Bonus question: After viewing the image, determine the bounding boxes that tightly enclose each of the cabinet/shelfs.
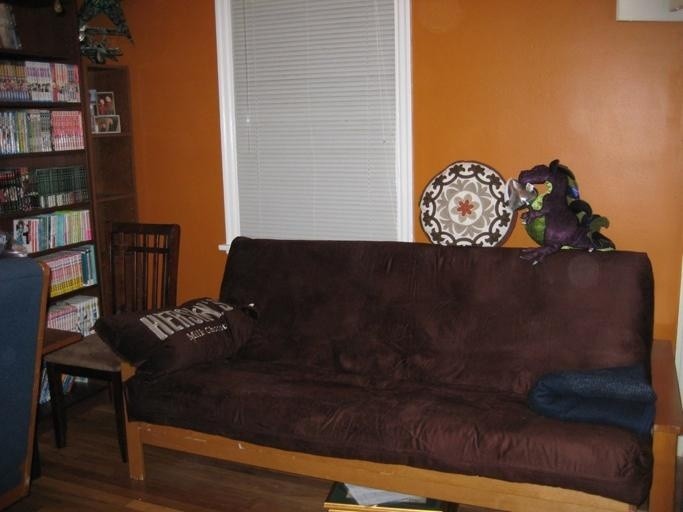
[0,49,109,424]
[83,60,147,385]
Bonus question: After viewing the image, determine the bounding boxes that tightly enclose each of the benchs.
[117,236,683,512]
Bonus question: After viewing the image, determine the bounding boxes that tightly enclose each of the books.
[0,59,103,404]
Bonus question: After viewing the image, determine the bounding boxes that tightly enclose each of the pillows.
[94,294,267,381]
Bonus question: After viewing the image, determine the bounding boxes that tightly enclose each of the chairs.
[42,221,180,448]
[1,253,52,511]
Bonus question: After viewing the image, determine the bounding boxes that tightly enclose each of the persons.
[99,95,115,131]
